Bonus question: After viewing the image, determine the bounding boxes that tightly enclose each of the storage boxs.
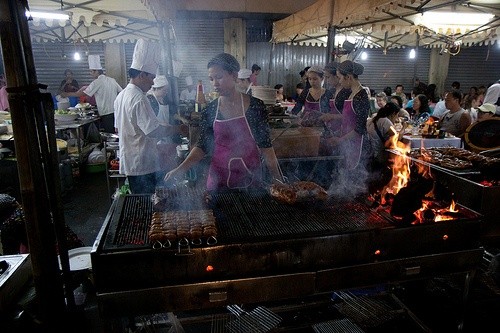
[269,127,324,159]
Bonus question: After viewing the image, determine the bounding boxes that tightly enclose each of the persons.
[59,69,81,93]
[180,75,199,103]
[274,83,285,102]
[250,64,262,86]
[289,66,329,121]
[300,62,348,157]
[318,59,371,184]
[358,77,500,183]
[63,54,127,152]
[165,52,284,192]
[236,68,256,96]
[146,73,194,145]
[115,37,192,195]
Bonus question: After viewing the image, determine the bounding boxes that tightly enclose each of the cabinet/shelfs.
[54,117,105,186]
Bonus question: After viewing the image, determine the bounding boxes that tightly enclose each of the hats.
[88,54,102,70]
[186,75,193,86]
[474,102,496,114]
[130,38,160,75]
[151,74,168,87]
[237,67,252,78]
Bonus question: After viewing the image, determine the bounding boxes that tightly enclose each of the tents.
[27,1,178,92]
[272,0,500,90]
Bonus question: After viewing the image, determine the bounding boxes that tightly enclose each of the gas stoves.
[0,253,34,321]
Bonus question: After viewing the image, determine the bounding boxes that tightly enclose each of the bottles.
[412,118,420,136]
[194,80,206,113]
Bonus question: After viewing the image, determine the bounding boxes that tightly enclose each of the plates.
[57,247,93,270]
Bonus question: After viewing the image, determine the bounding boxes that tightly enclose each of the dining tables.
[392,123,462,150]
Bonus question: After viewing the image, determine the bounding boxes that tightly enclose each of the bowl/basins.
[99,132,118,141]
[54,114,79,121]
[88,162,107,172]
[268,106,286,116]
